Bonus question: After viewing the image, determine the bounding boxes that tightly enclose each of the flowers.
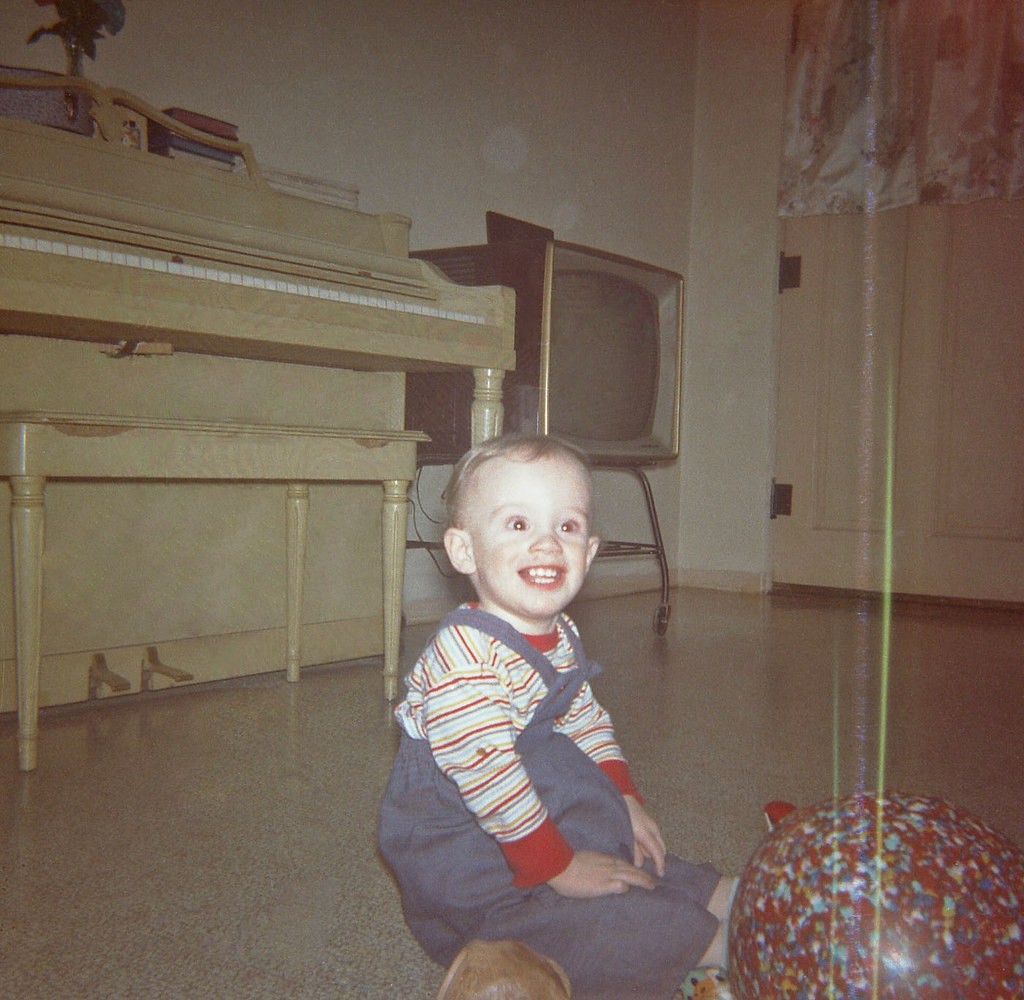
[28,0,127,60]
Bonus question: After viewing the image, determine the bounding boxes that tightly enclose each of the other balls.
[722,787,1023,1000]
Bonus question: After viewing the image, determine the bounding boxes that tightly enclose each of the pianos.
[0,71,517,773]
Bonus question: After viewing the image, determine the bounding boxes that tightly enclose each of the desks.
[401,454,671,637]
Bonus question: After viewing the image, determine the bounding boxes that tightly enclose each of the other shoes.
[762,800,796,831]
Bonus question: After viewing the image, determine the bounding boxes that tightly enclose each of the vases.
[63,42,88,77]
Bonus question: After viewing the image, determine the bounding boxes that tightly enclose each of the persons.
[372,431,738,1000]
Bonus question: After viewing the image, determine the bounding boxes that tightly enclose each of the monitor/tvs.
[405,238,686,462]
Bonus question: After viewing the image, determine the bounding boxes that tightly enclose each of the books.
[147,107,239,174]
[254,164,361,212]
